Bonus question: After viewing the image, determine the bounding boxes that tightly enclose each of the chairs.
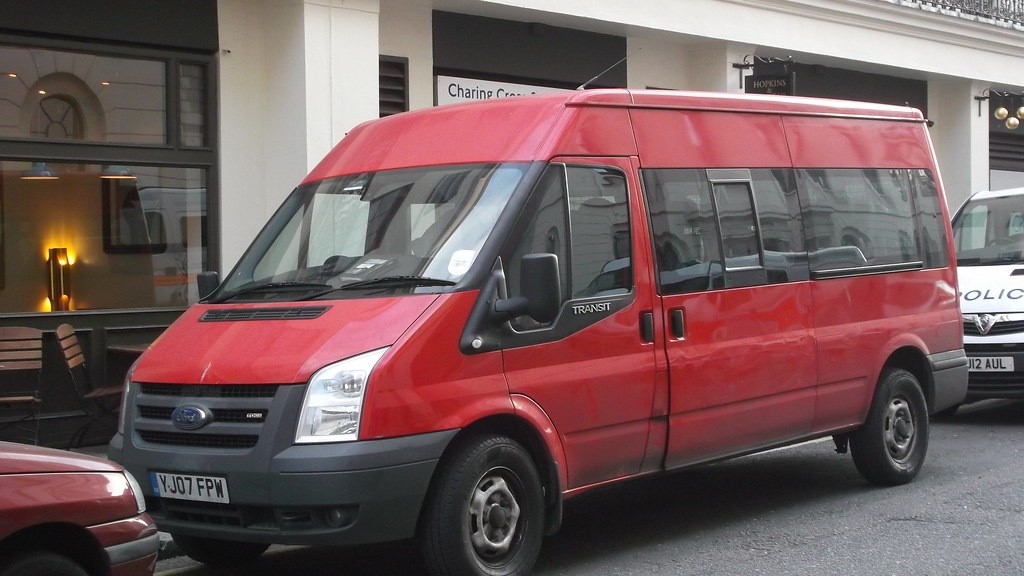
[0,327,43,445]
[574,196,617,290]
[55,323,124,451]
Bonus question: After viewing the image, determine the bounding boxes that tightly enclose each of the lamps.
[18,163,61,180]
[101,163,137,180]
[974,87,1024,130]
[47,247,72,311]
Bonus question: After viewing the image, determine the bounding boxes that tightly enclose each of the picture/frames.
[102,163,167,254]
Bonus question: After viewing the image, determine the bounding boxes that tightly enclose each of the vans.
[941,187,1024,423]
[104,85,974,576]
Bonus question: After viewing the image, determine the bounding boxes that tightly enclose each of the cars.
[0,439,162,576]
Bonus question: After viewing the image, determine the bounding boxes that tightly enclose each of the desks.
[109,344,148,354]
